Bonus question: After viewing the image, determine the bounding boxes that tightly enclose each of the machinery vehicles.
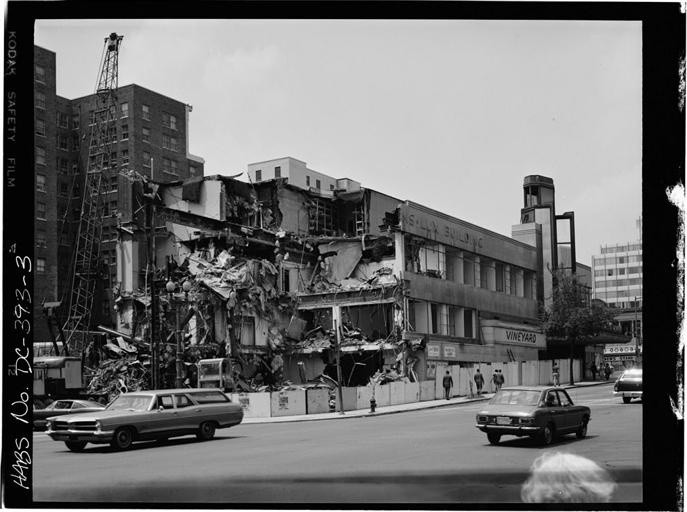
[33,33,123,403]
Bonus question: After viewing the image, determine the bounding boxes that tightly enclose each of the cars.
[47,386,245,453]
[474,384,592,448]
[32,398,106,434]
[612,370,643,404]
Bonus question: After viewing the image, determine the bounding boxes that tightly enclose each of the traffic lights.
[329,331,336,354]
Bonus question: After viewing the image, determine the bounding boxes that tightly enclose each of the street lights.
[164,279,193,391]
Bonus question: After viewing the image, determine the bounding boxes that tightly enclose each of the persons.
[474,369,484,394]
[591,361,611,381]
[442,370,453,400]
[552,363,560,387]
[490,369,504,392]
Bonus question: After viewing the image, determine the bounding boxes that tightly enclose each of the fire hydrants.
[369,398,377,412]
